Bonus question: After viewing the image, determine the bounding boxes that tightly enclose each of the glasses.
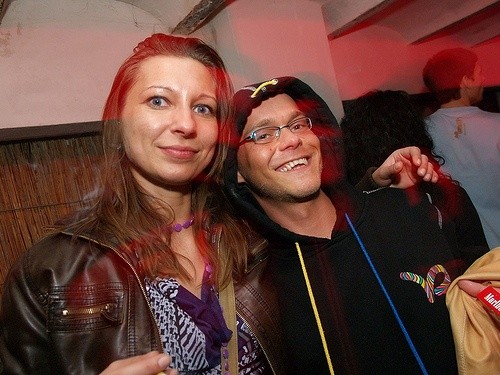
[236,116,312,149]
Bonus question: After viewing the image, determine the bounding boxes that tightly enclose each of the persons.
[224,76,500,375]
[0,34,438,375]
[423,49,500,250]
[339,88,445,172]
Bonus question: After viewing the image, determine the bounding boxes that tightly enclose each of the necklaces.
[172,219,194,232]
